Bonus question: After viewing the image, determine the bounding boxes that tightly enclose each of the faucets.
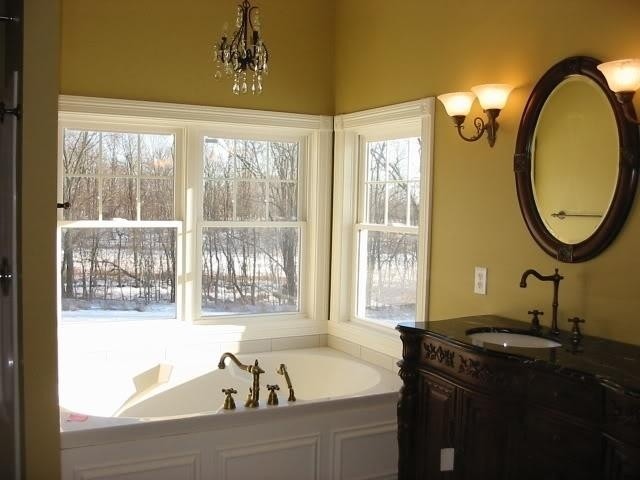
[218,353,265,408]
[277,364,296,402]
[519,268,565,334]
[466,327,563,349]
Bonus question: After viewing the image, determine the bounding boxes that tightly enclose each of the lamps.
[208,0,272,96]
[437,84,513,148]
[597,58,640,124]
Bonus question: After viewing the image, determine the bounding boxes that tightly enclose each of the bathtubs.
[57,347,404,480]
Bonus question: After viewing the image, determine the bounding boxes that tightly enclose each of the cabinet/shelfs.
[397,326,640,480]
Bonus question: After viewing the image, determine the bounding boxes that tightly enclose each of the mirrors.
[514,56,639,264]
